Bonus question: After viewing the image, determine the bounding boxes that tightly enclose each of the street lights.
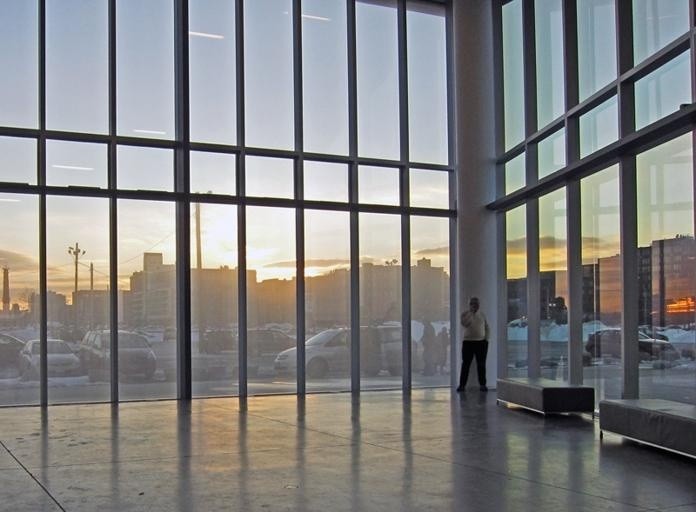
[385,258,398,266]
[68,243,86,320]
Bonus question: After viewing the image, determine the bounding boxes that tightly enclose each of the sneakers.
[480,386,488,392]
[457,386,464,392]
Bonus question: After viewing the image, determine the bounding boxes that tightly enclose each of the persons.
[436,326,450,375]
[547,295,570,382]
[456,297,491,392]
[420,319,437,377]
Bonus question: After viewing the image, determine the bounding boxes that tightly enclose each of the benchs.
[496,377,595,419]
[599,399,696,459]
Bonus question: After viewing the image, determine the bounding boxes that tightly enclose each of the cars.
[585,326,676,360]
